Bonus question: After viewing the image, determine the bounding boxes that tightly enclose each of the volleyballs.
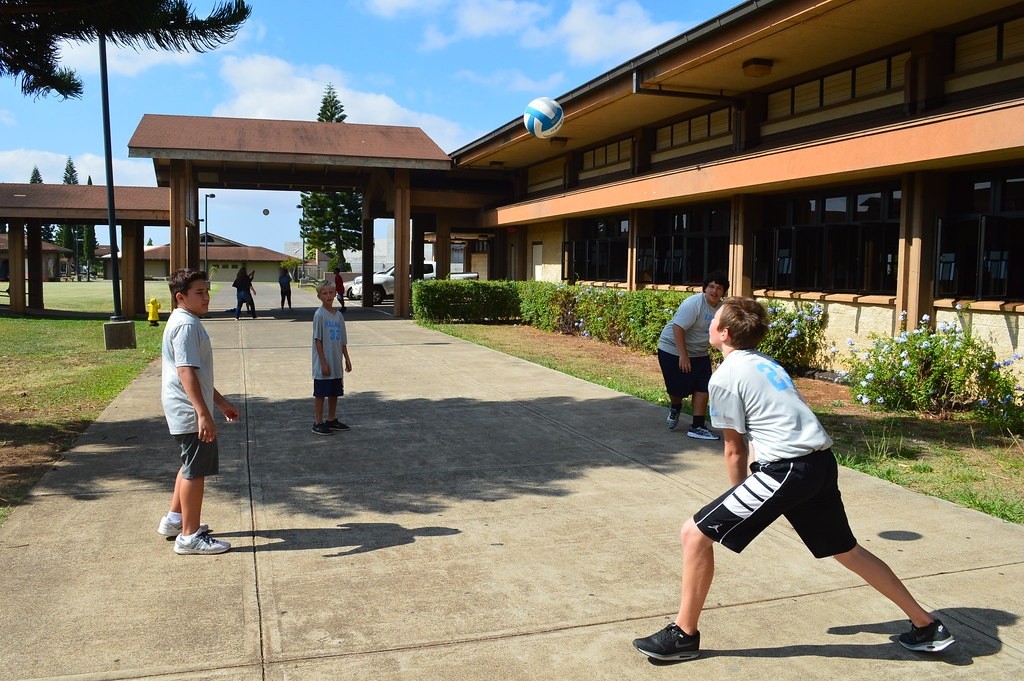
[523,97,565,140]
[263,209,269,215]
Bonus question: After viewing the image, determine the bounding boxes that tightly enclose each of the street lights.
[204,193,215,291]
[296,204,305,279]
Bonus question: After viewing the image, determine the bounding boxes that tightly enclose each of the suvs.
[339,259,436,306]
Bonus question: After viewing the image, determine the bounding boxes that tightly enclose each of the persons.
[235,265,257,321]
[310,280,352,435]
[278,266,293,311]
[657,272,730,440]
[333,267,347,313]
[156,267,239,554]
[632,293,955,662]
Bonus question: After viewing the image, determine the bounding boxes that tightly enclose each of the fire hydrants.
[146,297,161,326]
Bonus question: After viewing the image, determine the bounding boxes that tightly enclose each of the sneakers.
[312,422,334,435]
[173,528,231,554]
[326,418,350,431]
[666,403,682,430]
[633,621,701,660]
[687,425,720,440]
[899,619,956,652]
[158,516,208,536]
